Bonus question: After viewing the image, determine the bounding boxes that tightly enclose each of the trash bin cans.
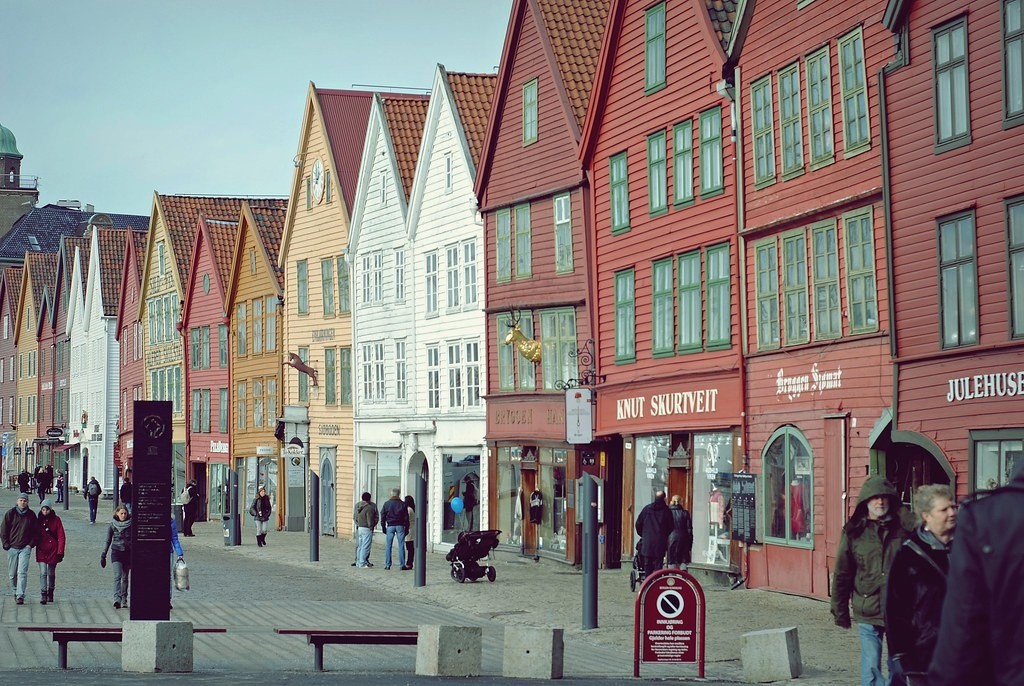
[223,514,242,545]
[170,504,184,532]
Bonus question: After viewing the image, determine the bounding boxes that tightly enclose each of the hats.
[40,498,53,509]
[17,493,29,500]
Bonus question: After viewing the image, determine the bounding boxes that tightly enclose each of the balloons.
[451,497,464,514]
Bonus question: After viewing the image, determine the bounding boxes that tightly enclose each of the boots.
[255,533,267,547]
[47,587,55,602]
[40,591,48,604]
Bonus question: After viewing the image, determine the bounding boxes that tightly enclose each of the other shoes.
[384,567,390,570]
[122,603,127,608]
[405,564,412,569]
[114,600,121,609]
[16,598,24,605]
[400,566,409,570]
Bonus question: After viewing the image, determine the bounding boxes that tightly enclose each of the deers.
[503,306,541,363]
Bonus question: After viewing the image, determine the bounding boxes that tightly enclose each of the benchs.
[18,626,227,669]
[272,626,418,673]
[103,487,121,499]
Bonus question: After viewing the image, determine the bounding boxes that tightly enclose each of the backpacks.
[88,484,98,496]
[181,486,193,504]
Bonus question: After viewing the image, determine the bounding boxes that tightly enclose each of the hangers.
[530,487,544,497]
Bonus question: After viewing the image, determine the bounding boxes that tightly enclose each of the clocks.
[311,156,325,205]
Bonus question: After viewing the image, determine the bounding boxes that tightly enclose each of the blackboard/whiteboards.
[731,473,761,546]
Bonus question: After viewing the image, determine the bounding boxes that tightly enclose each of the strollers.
[450,529,502,583]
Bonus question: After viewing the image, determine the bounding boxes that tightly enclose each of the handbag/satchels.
[173,557,190,591]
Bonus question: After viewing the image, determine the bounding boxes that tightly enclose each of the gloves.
[894,658,906,685]
[382,527,387,534]
[404,529,409,535]
[100,553,106,568]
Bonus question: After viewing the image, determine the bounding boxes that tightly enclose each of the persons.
[668,495,693,580]
[249,488,272,547]
[886,484,957,686]
[710,488,724,537]
[18,465,64,504]
[101,478,183,609]
[780,480,810,540]
[831,476,916,686]
[84,477,102,524]
[635,490,674,588]
[0,493,37,604]
[32,499,66,604]
[351,488,416,570]
[926,462,1024,686]
[462,475,476,533]
[180,479,199,536]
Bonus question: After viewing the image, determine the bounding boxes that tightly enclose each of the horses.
[281,353,318,386]
